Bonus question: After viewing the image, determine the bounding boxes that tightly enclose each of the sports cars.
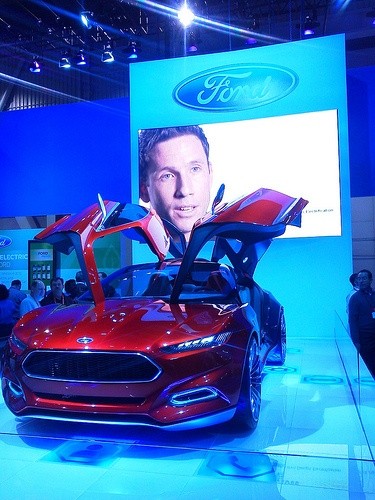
[2,188,287,431]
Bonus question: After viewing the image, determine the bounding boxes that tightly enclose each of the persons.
[65,270,107,299]
[0,284,16,328]
[40,277,74,305]
[345,269,375,376]
[7,280,27,304]
[138,125,211,242]
[20,280,45,317]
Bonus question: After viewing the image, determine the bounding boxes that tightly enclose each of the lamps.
[304,15,315,35]
[187,30,199,52]
[127,41,138,59]
[29,55,41,73]
[76,48,86,65]
[245,14,258,44]
[58,49,71,69]
[101,42,114,63]
[80,11,94,28]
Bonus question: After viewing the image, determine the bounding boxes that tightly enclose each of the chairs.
[142,271,173,297]
[204,272,227,292]
